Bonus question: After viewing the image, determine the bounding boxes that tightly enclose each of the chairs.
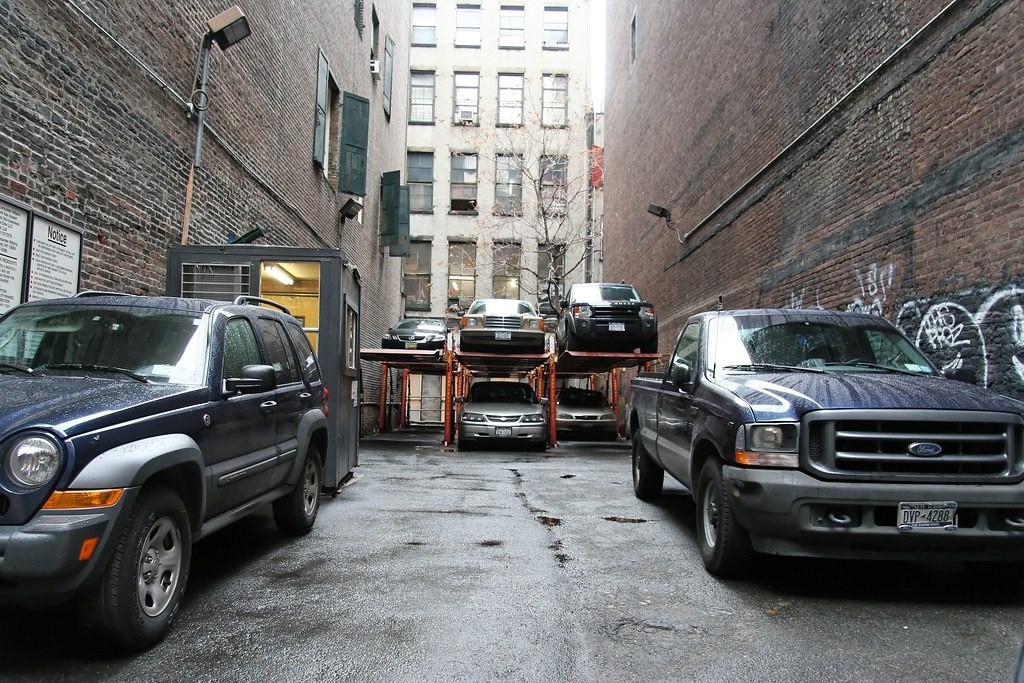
[805,345,841,365]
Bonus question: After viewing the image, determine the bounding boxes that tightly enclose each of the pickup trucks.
[628,307,1024,580]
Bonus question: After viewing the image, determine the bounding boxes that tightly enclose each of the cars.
[454,380,550,452]
[456,298,548,355]
[544,386,619,441]
[382,316,451,351]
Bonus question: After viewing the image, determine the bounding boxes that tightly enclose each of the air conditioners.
[459,110,474,120]
[543,40,558,48]
[370,58,380,80]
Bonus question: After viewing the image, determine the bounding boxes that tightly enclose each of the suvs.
[555,280,659,357]
[0,289,332,651]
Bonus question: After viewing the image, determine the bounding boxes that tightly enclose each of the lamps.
[338,198,363,224]
[647,203,671,222]
[263,263,296,285]
[204,5,252,51]
